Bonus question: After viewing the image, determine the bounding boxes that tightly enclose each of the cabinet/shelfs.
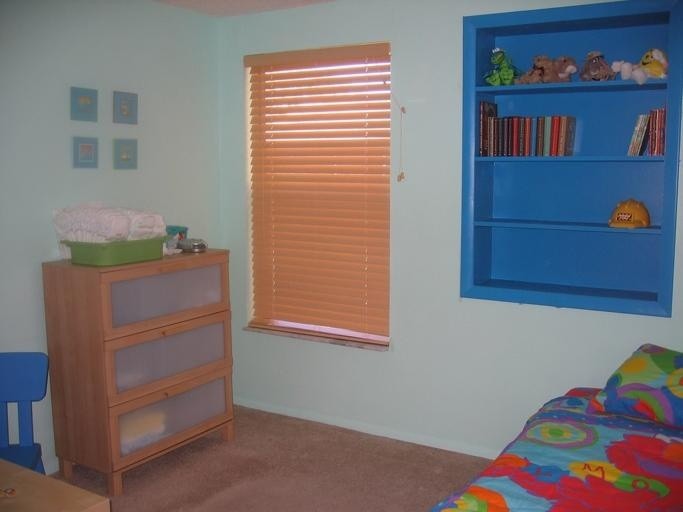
[460,0,682,318]
[41,249,235,497]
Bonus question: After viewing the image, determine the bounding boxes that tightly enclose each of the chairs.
[0,353,48,474]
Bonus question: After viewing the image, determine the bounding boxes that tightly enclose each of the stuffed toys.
[484,44,668,86]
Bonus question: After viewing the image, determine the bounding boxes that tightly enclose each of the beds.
[428,388,683,512]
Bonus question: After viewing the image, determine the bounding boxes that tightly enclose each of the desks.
[0,457,111,512]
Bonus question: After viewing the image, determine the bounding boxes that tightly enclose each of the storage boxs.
[61,236,171,265]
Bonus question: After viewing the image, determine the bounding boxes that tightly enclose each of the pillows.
[586,343,683,431]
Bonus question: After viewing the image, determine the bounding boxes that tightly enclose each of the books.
[478,101,576,157]
[623,108,665,156]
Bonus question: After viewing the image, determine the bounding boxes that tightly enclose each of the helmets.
[608,198,651,228]
